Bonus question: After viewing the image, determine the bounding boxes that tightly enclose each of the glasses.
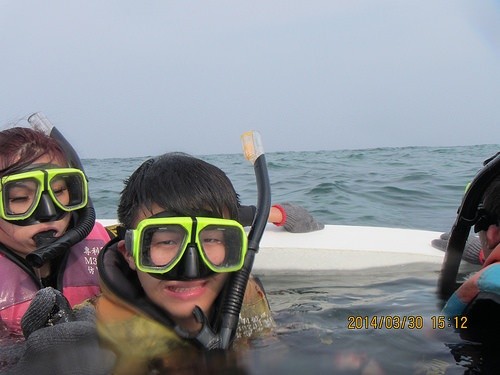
[1,163,88,226]
[121,206,248,281]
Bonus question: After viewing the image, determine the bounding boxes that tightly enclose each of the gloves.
[271,201,325,233]
[431,231,481,266]
[0,286,119,375]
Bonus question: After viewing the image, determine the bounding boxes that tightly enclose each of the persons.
[437,166,500,375]
[0,112,326,375]
[73,151,282,375]
